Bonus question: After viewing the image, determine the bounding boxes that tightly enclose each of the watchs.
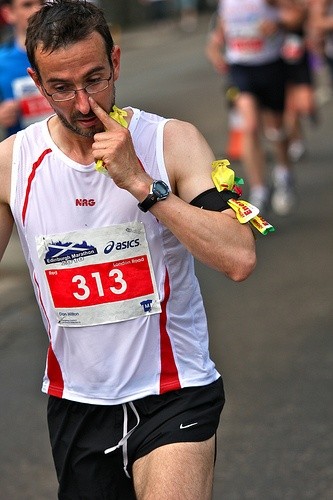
[136,180,169,214]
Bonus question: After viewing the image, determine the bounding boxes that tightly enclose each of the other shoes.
[249,163,298,224]
[299,105,321,131]
[286,135,310,164]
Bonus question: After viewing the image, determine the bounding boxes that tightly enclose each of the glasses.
[10,0,47,11]
[34,53,114,103]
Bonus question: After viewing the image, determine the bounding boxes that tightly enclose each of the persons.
[304,0,332,109]
[203,0,297,218]
[0,0,257,500]
[0,0,56,139]
[264,0,321,164]
